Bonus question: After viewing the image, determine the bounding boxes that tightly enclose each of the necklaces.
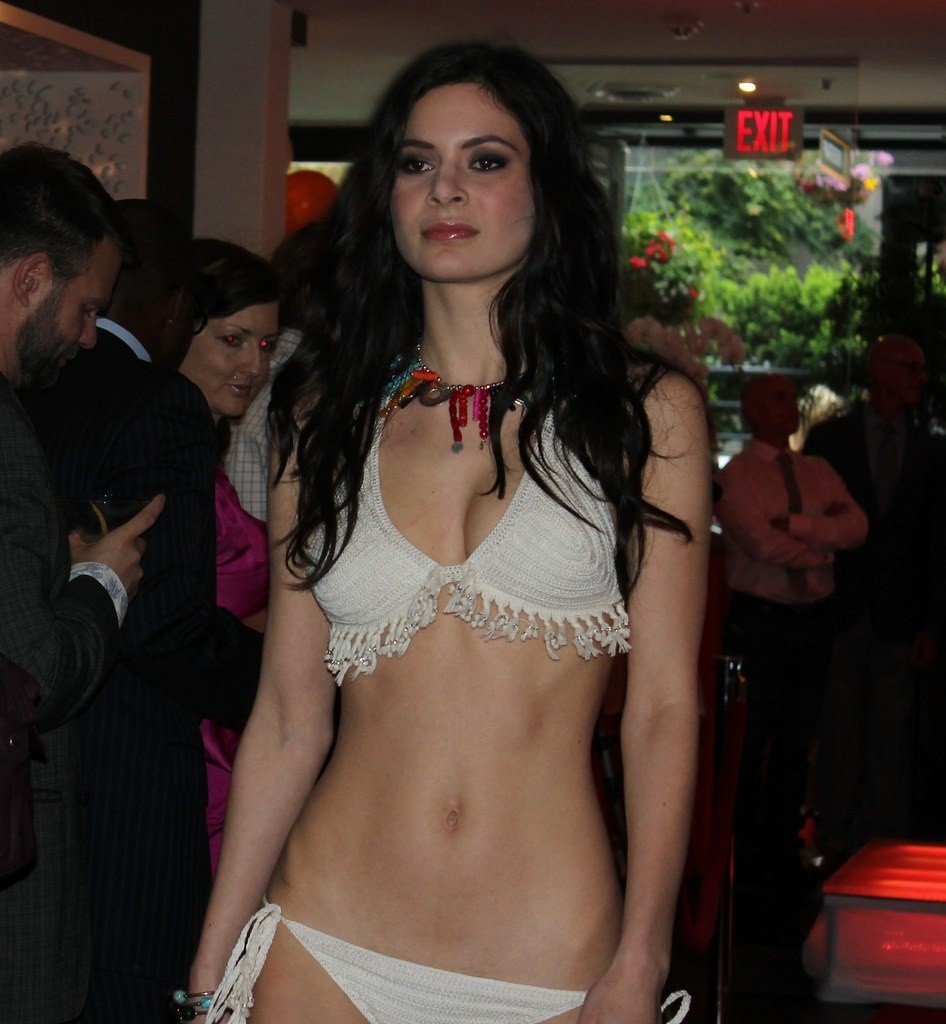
[378,338,533,452]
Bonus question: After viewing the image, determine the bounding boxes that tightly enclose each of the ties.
[876,424,894,521]
[777,452,808,595]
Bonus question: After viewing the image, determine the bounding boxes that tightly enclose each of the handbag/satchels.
[0,652,50,883]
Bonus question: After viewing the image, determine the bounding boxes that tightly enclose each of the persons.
[191,39,713,1024]
[0,142,946,1024]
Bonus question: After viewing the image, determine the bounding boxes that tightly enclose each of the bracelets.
[173,991,216,1003]
[176,1006,210,1021]
[172,998,213,1014]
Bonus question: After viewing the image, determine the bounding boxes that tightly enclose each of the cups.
[66,496,155,542]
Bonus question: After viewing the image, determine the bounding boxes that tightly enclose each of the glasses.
[167,283,208,335]
[885,357,930,375]
